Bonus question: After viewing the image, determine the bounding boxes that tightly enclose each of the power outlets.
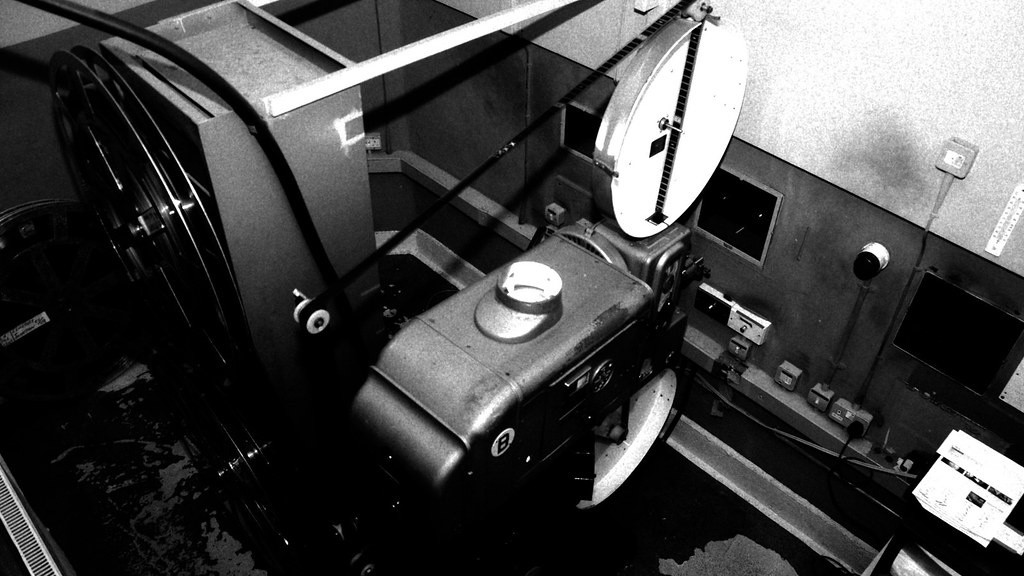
[828,398,874,439]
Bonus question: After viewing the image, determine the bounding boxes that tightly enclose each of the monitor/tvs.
[891,272,1024,396]
[693,166,785,269]
